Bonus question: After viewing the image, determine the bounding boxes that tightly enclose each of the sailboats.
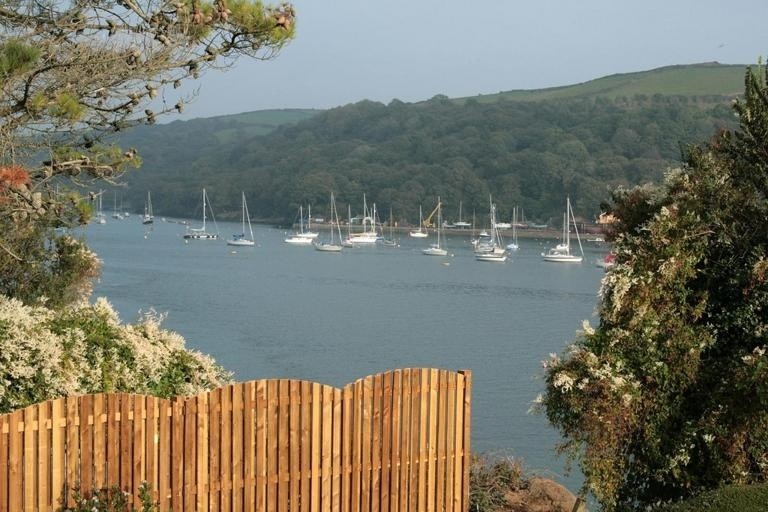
[284,190,397,251]
[408,192,549,261]
[96,183,221,241]
[540,194,587,263]
[226,190,255,246]
